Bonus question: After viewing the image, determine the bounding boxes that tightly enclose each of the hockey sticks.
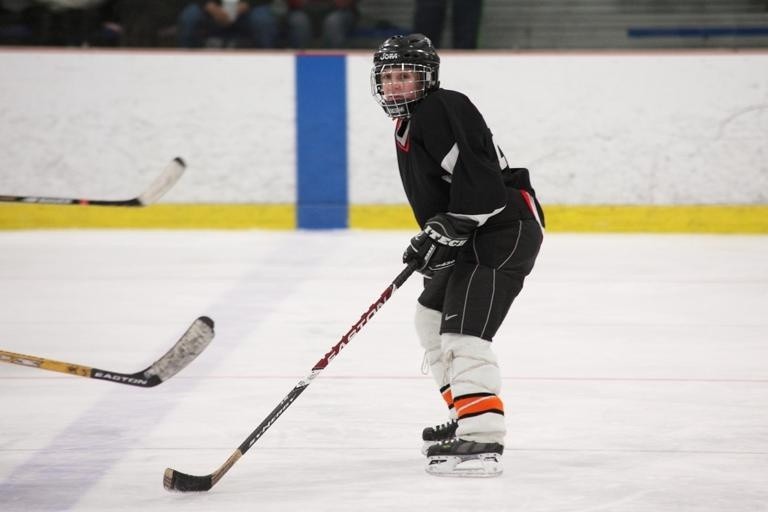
[0,157,185,207]
[1,316,215,386]
[163,262,421,493]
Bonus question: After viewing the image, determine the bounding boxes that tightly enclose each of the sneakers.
[427,436,504,456]
[422,417,461,440]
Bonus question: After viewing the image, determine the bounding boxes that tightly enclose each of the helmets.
[370,35,440,121]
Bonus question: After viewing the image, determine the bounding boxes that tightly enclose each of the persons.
[371,35,545,457]
[2,0,362,50]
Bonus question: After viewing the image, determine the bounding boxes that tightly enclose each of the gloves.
[402,213,479,280]
